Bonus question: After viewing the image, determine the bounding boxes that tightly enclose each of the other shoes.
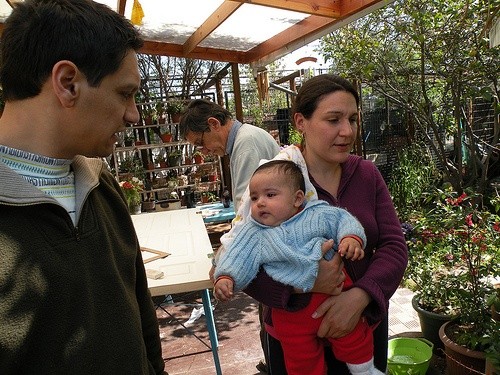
[259,359,268,373]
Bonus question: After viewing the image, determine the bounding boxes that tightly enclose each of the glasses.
[195,128,205,151]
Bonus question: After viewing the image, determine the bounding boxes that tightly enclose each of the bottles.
[223,187,230,208]
[186,187,195,208]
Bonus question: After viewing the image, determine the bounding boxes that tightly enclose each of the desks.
[194,200,237,235]
[131,208,223,375]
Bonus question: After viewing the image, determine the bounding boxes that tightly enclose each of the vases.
[412,294,455,358]
[222,186,231,208]
[132,205,141,215]
[437,321,487,375]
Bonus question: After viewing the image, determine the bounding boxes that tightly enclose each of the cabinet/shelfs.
[116,98,227,194]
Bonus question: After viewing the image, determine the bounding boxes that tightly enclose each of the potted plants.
[112,92,217,211]
[288,123,304,153]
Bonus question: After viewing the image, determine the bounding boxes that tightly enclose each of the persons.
[179,99,279,375]
[214,160,386,375]
[221,74,408,375]
[0,0,169,375]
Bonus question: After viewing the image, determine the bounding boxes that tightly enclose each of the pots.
[387,337,434,375]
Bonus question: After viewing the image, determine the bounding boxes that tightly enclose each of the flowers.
[402,191,500,375]
[117,176,144,213]
